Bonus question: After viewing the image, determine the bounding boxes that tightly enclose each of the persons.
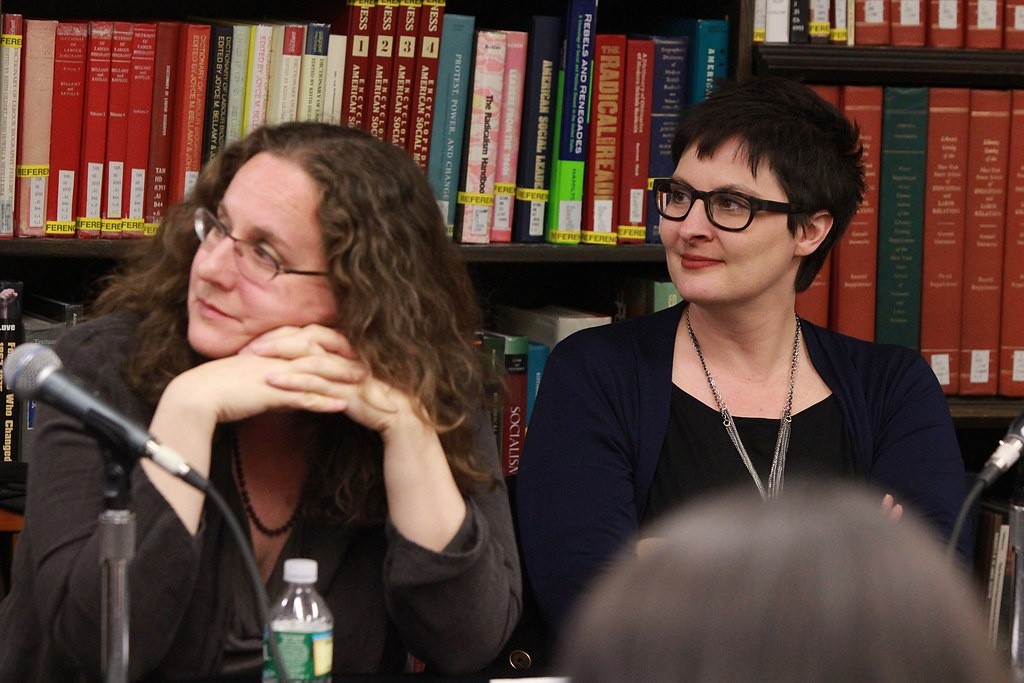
[0,120,522,683]
[562,475,1007,683]
[515,79,1010,683]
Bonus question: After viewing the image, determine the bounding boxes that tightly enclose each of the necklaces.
[684,306,799,505]
[228,420,297,535]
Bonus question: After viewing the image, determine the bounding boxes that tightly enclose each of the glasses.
[193,206,333,285]
[648,177,805,233]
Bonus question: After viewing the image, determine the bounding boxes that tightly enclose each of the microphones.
[4,342,212,492]
[980,416,1024,485]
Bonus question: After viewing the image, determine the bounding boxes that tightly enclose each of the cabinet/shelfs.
[0,0,1024,421]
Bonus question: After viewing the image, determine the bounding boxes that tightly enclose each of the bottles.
[262,559,333,682]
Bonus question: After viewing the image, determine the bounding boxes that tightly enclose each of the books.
[793,76,1024,397]
[0,280,103,515]
[472,291,612,476]
[976,497,1024,683]
[0,11,728,247]
[753,0,1024,52]
